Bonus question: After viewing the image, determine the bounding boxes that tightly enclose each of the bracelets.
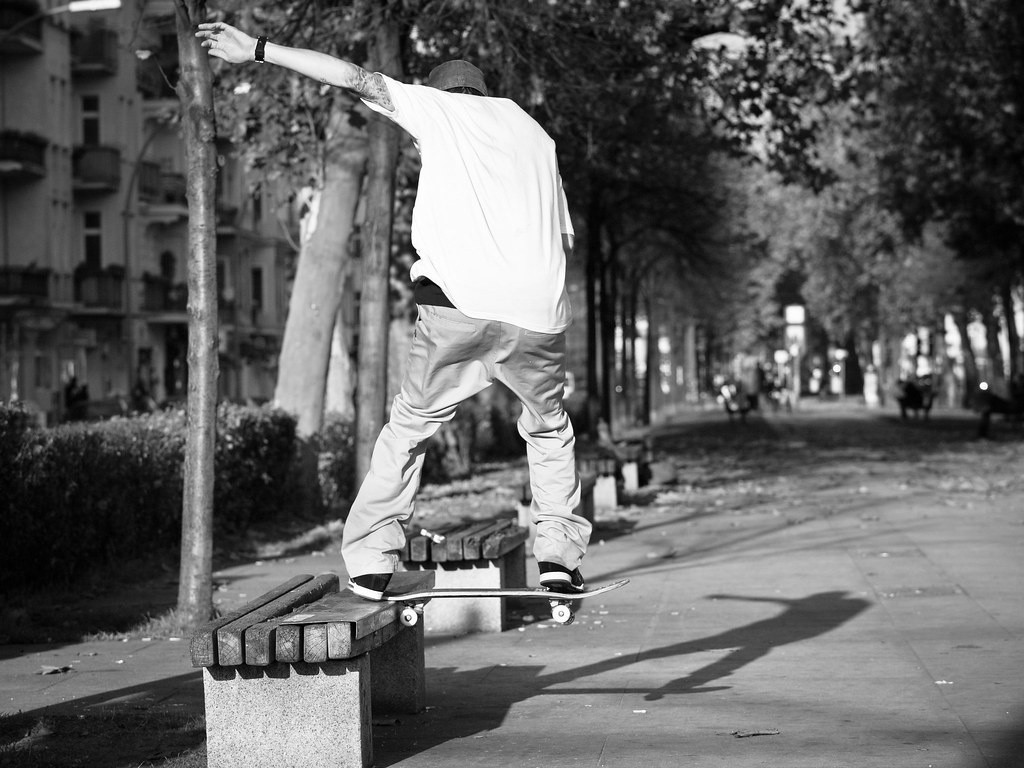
[255,35,269,63]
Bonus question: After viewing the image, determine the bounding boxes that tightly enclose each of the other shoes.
[346,573,392,602]
[537,562,585,592]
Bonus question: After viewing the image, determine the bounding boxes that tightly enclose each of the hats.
[427,59,489,96]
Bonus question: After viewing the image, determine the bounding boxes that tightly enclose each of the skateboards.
[381,577,630,628]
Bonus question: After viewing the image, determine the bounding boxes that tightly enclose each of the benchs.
[403,519,532,632]
[615,449,645,490]
[579,459,623,508]
[191,570,437,768]
[513,472,598,548]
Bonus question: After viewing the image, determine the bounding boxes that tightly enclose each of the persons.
[195,22,594,600]
[64,375,88,421]
[138,356,186,401]
